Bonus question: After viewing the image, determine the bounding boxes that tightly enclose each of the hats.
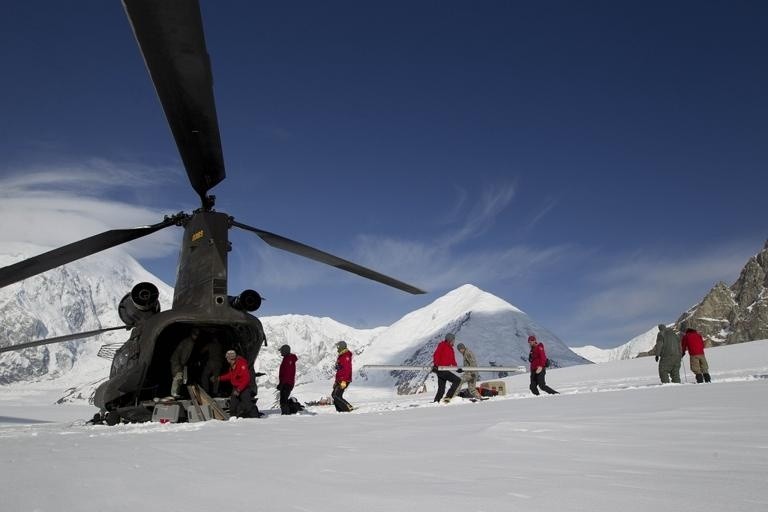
[457,343,465,350]
[336,341,347,350]
[445,333,455,343]
[226,350,236,359]
[278,345,289,354]
[528,336,536,342]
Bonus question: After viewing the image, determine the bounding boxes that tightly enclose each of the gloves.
[458,368,462,372]
[432,366,438,372]
[536,367,542,374]
[341,381,347,390]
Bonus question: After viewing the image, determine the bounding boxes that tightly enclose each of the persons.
[452,343,482,399]
[277,344,300,415]
[528,336,560,395]
[200,339,222,396]
[681,328,711,383]
[655,324,681,383]
[330,341,355,412]
[171,327,199,398]
[432,333,461,403]
[211,350,262,421]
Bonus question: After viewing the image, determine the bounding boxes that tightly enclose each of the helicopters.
[0,0,428,427]
